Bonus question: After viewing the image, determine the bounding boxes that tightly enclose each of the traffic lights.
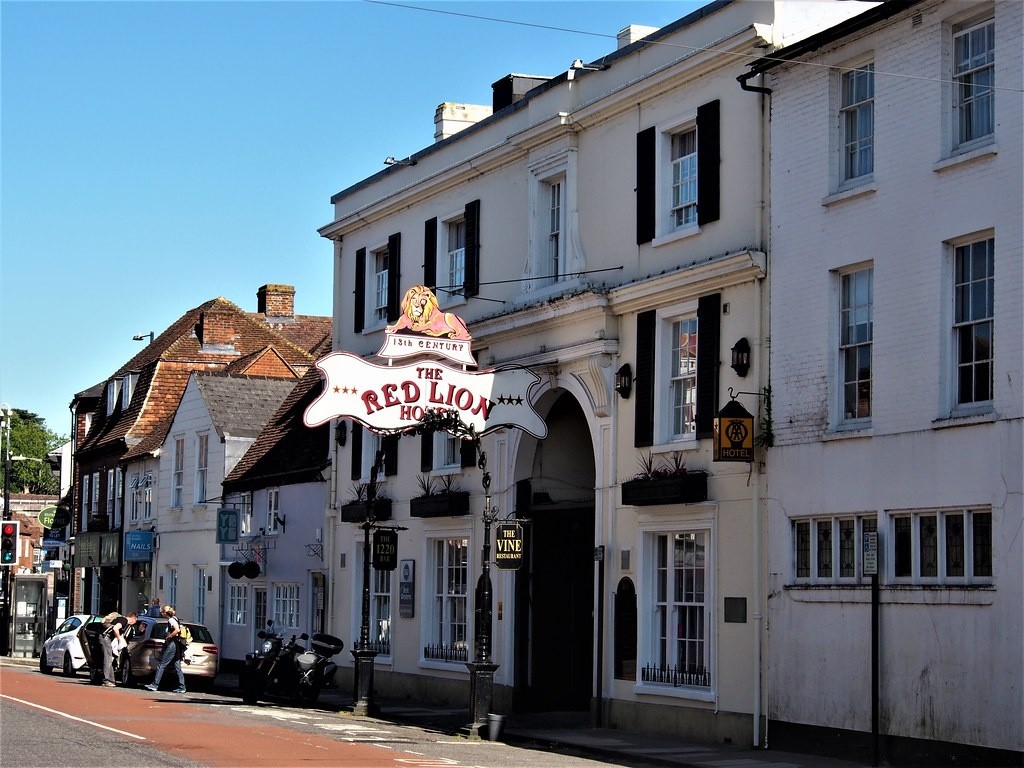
[0,521,21,567]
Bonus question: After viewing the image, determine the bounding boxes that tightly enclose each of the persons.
[99,597,186,694]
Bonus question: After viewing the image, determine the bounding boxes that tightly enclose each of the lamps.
[712,387,767,462]
[614,363,633,399]
[730,337,751,377]
[335,420,347,446]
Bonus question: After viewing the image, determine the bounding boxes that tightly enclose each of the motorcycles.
[238,619,344,706]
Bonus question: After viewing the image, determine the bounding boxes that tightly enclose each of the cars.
[80,613,219,684]
[38,613,103,674]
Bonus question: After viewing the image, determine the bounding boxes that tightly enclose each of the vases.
[410,495,470,518]
[622,473,709,506]
[342,503,392,522]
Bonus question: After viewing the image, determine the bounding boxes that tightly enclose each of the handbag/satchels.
[180,625,193,647]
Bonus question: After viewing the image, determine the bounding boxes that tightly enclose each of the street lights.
[0,456,26,653]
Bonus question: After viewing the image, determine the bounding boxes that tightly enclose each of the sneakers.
[144,684,158,691]
[102,681,116,687]
[173,688,186,693]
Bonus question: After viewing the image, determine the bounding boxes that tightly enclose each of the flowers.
[410,471,470,498]
[343,482,393,505]
[634,449,688,479]
